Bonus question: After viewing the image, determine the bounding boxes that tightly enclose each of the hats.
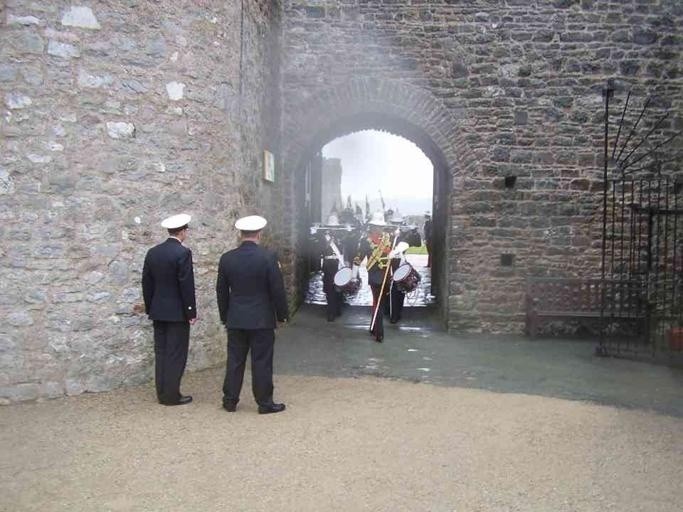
[322,209,431,228]
[161,213,191,230]
[234,215,267,232]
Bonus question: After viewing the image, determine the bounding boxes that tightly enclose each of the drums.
[334,265,362,295]
[393,263,421,293]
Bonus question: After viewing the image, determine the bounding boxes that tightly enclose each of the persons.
[140,212,196,404]
[315,210,432,343]
[215,215,284,412]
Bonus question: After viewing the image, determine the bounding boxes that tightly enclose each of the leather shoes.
[159,395,192,406]
[258,403,285,414]
[325,309,402,343]
